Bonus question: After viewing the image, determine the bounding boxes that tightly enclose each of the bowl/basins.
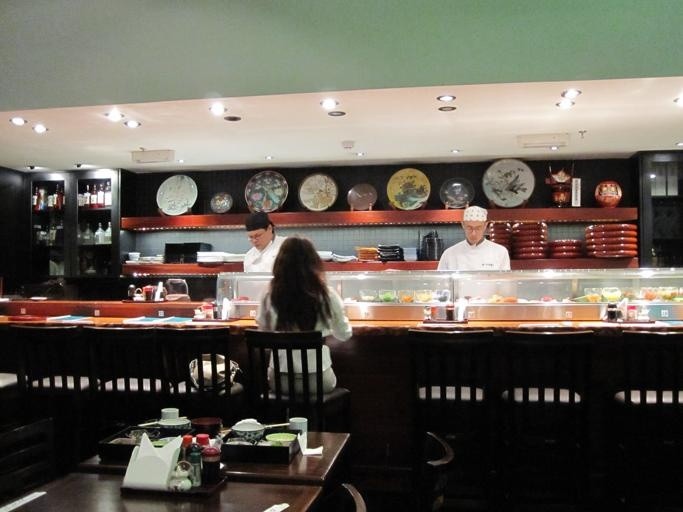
[158,419,191,430]
[191,417,221,434]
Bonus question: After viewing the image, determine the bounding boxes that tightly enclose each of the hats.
[246,212,270,230]
[464,206,487,221]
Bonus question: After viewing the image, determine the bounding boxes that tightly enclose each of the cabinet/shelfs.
[120,205,639,277]
[24,169,134,300]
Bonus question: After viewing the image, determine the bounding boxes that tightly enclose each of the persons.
[242,211,286,274]
[256,238,352,393]
[437,205,512,298]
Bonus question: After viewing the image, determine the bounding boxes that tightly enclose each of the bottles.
[127,284,168,302]
[422,304,455,322]
[180,431,222,487]
[607,303,636,323]
[31,182,113,246]
[201,301,220,320]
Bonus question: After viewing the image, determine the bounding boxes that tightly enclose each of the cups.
[583,286,683,304]
[160,407,180,420]
[228,425,297,446]
[358,289,451,304]
[425,238,443,261]
[128,252,140,261]
[165,278,188,295]
[290,417,309,451]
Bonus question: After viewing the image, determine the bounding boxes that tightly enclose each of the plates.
[347,183,378,211]
[440,178,476,209]
[482,158,537,209]
[163,242,244,264]
[317,245,404,263]
[298,173,338,212]
[244,170,289,213]
[386,167,432,210]
[125,260,138,264]
[486,217,639,259]
[210,192,233,214]
[156,175,199,216]
